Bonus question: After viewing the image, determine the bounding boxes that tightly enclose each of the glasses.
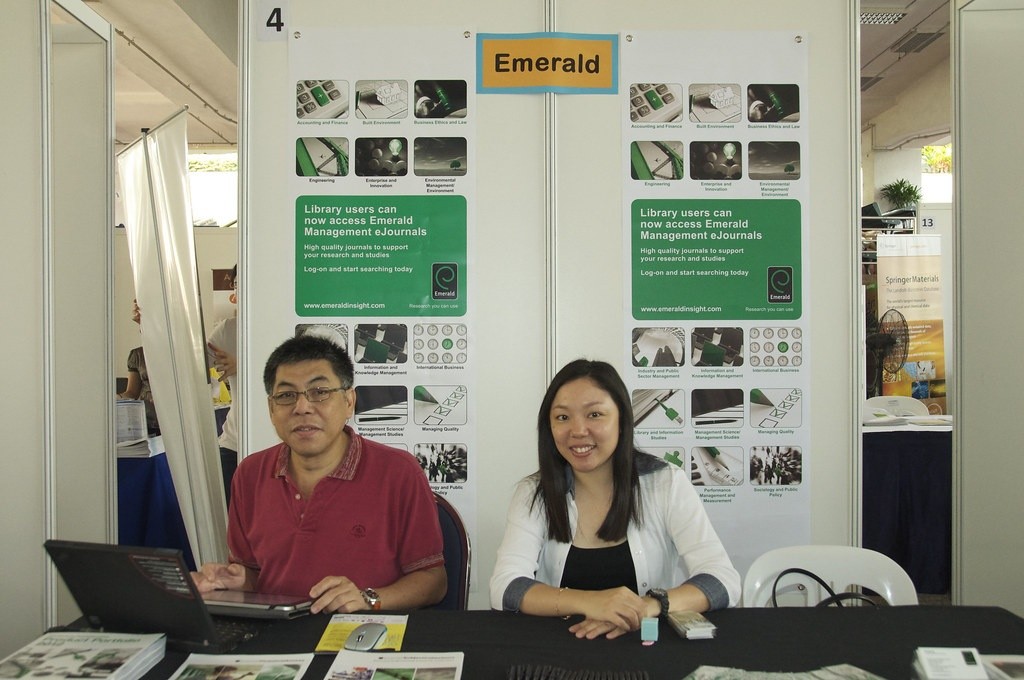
[271,387,346,406]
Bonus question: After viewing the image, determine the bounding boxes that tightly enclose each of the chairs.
[744,547,919,606]
[431,492,473,609]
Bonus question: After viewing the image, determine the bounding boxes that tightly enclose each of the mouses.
[344,623,387,650]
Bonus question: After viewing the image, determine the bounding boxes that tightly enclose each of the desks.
[41,608,1024,680]
[858,425,952,595]
[114,407,235,571]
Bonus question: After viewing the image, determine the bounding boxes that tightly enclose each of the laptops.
[42,539,258,654]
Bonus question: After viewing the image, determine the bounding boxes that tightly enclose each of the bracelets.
[555,587,571,620]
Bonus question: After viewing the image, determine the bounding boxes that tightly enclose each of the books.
[114,435,166,458]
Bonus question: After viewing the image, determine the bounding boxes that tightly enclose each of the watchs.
[646,587,669,617]
[360,588,381,610]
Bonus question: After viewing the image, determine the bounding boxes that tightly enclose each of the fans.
[867,307,911,397]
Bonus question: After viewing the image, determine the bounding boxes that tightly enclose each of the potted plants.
[880,179,923,217]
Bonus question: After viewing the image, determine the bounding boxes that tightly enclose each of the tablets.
[200,590,312,620]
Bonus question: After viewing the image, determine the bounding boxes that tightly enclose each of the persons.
[190,337,448,614]
[115,346,160,435]
[131,263,237,491]
[489,360,742,639]
